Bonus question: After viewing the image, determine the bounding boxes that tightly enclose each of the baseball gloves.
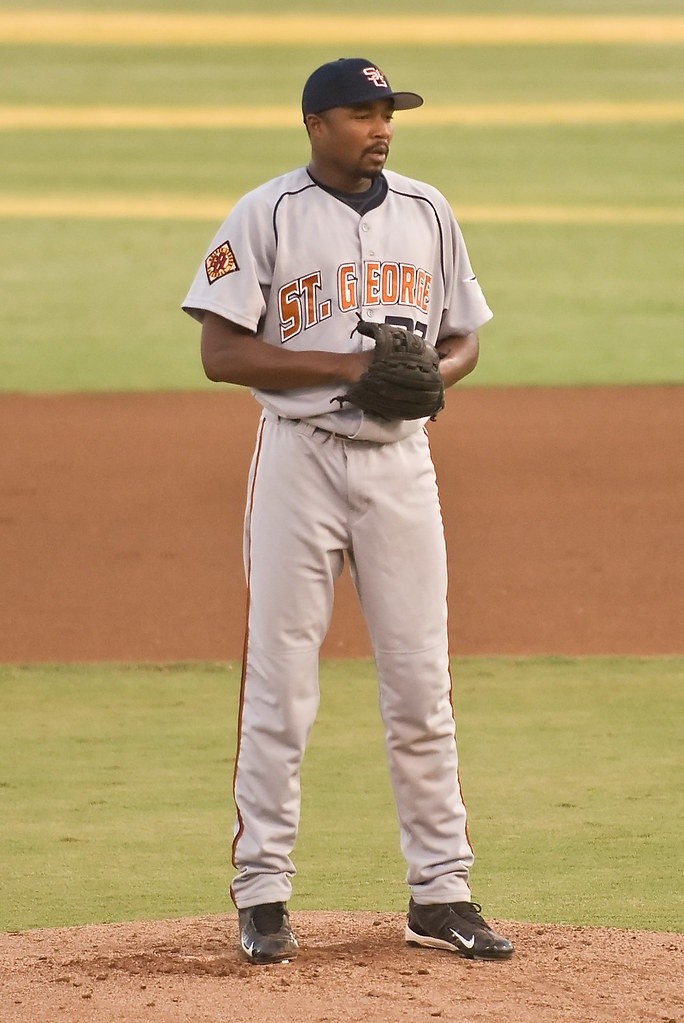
[346,319,444,421]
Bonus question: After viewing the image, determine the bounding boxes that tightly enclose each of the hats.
[301,56,424,121]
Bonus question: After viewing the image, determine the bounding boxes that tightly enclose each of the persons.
[182,58,515,965]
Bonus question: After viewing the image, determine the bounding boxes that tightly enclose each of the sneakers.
[238,901,300,965]
[405,895,515,962]
